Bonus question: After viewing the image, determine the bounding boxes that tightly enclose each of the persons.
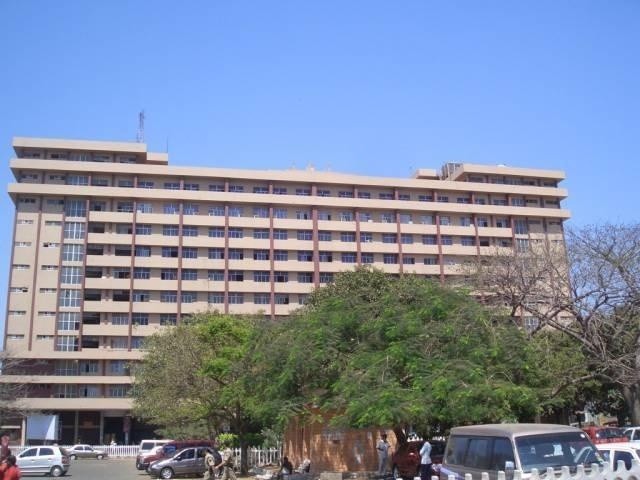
[278,457,292,475]
[419,435,433,480]
[0,433,12,466]
[376,434,390,478]
[215,441,237,480]
[203,448,217,480]
[0,455,20,480]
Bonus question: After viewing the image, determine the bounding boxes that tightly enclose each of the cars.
[392,439,446,480]
[579,419,640,471]
[65,443,108,461]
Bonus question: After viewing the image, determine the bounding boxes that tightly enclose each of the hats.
[221,440,230,445]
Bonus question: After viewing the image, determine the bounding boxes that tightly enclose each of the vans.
[440,422,610,480]
[14,444,69,477]
[136,439,223,480]
